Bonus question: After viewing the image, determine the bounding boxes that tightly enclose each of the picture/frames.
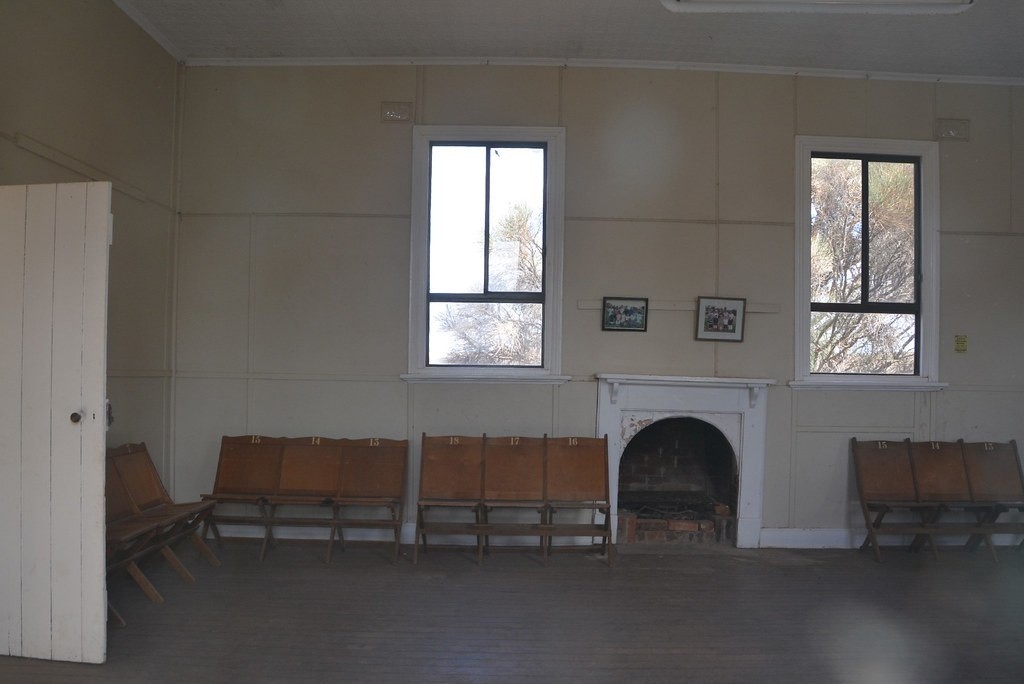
[600,297,649,333]
[695,296,747,343]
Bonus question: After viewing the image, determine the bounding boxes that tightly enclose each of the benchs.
[850,436,1024,565]
[412,433,615,566]
[196,434,409,565]
[105,440,221,627]
